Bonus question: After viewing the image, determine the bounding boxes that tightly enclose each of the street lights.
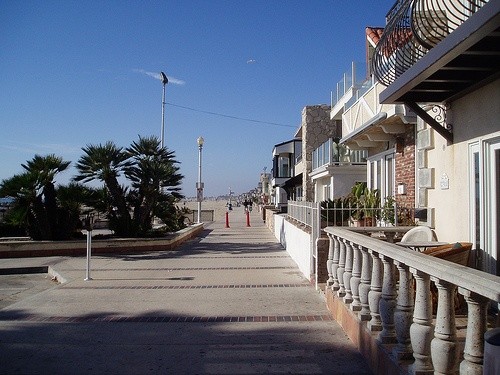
[196,137,205,222]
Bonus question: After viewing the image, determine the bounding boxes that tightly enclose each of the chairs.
[414,242,473,310]
[401,226,433,252]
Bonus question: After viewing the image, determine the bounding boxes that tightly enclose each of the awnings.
[379,0,500,143]
[280,173,302,194]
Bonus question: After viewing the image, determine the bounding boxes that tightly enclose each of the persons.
[248,199,253,211]
[244,200,249,214]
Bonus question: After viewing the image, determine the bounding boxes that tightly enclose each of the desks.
[396,242,449,252]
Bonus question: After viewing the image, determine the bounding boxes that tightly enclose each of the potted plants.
[348,182,395,227]
